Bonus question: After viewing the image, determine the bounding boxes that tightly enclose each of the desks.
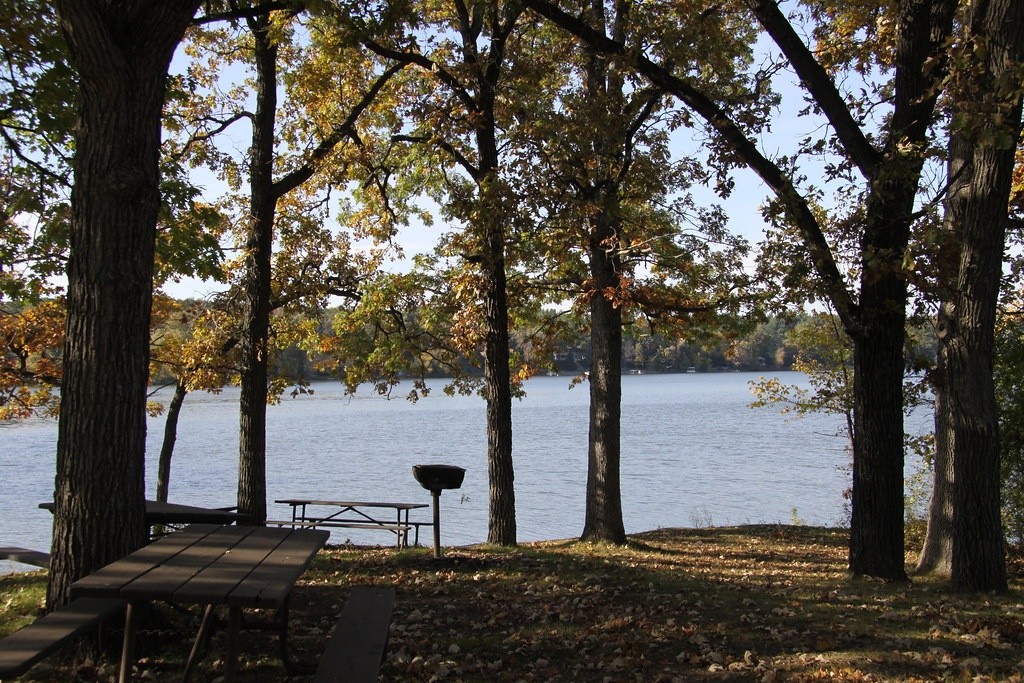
[275,499,430,548]
[37,500,250,546]
[66,522,331,683]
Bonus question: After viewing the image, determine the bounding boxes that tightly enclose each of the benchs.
[314,584,397,683]
[0,546,53,570]
[266,517,433,553]
[0,597,127,682]
